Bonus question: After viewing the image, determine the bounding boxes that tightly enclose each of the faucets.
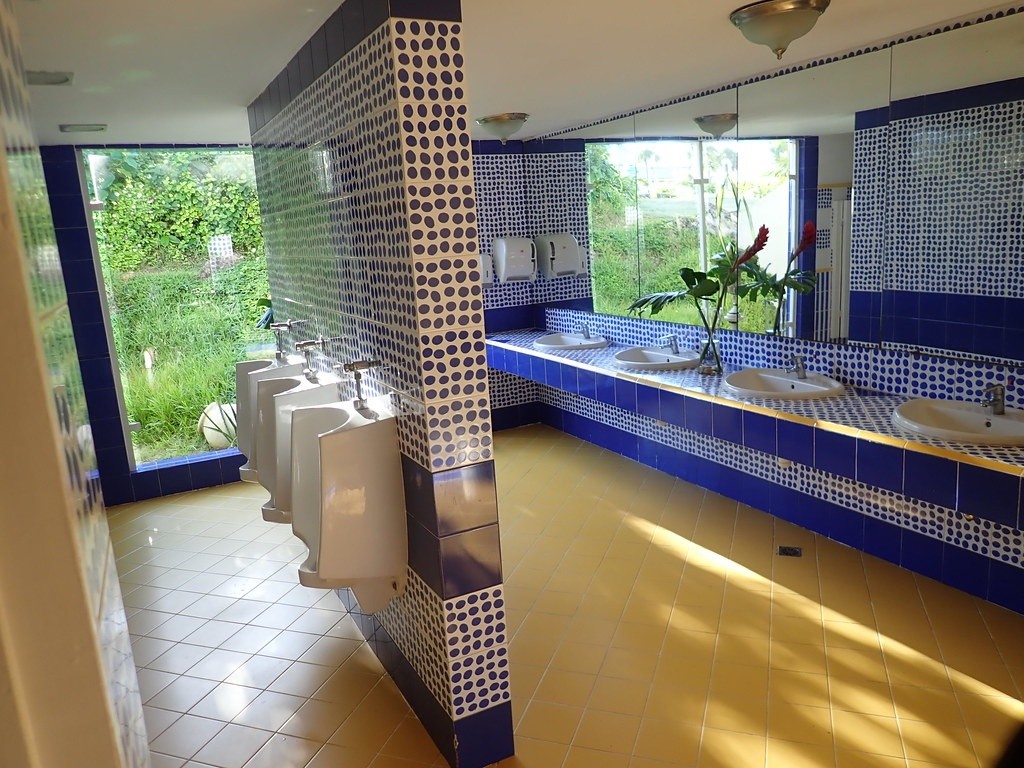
[784,355,807,379]
[574,322,591,339]
[980,383,1006,416]
[659,333,680,354]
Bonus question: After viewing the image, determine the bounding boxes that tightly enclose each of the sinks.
[612,346,701,370]
[533,332,608,349]
[892,397,1024,445]
[724,367,845,398]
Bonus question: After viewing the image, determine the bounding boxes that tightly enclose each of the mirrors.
[522,11,1023,368]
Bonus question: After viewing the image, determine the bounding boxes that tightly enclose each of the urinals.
[234,318,408,618]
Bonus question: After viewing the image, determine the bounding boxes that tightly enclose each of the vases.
[765,298,788,338]
[699,310,723,376]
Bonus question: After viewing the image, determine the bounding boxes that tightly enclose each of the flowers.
[734,220,820,338]
[627,185,770,370]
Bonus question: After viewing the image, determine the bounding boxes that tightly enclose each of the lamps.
[478,111,528,147]
[694,113,738,141]
[728,0,832,60]
